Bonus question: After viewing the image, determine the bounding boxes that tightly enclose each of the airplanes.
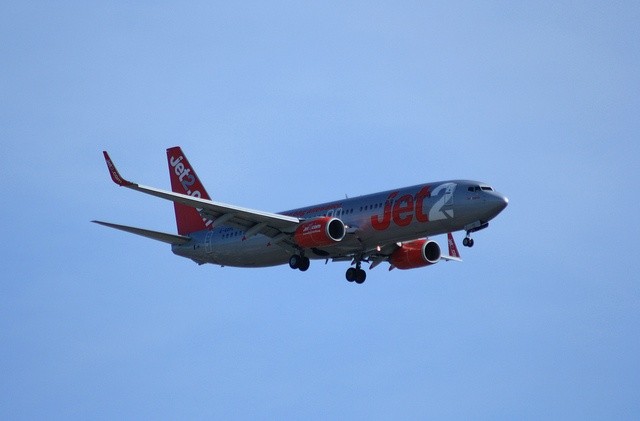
[92,141,512,286]
